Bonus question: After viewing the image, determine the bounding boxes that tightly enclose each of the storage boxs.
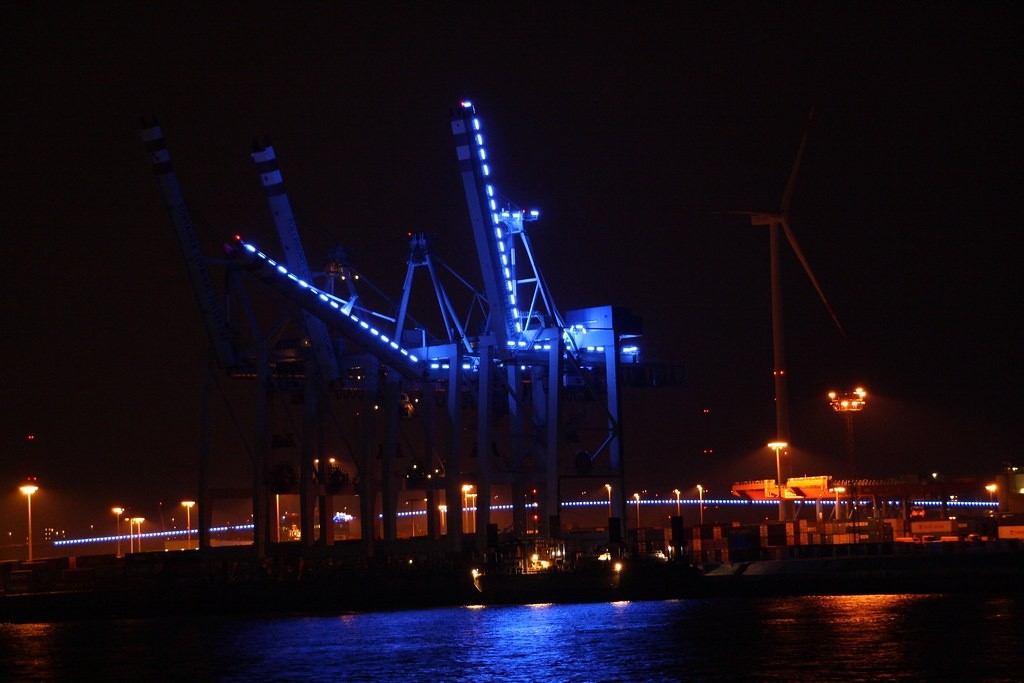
[635,518,1024,564]
[0,550,226,596]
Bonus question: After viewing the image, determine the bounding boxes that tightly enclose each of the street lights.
[462,485,473,533]
[439,505,447,525]
[834,487,845,518]
[697,484,703,525]
[181,500,196,548]
[675,489,680,516]
[467,494,477,533]
[18,484,39,561]
[112,507,125,555]
[634,493,640,532]
[768,442,788,500]
[605,484,612,516]
[132,517,145,552]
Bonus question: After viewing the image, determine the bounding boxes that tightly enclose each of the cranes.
[146,93,643,591]
